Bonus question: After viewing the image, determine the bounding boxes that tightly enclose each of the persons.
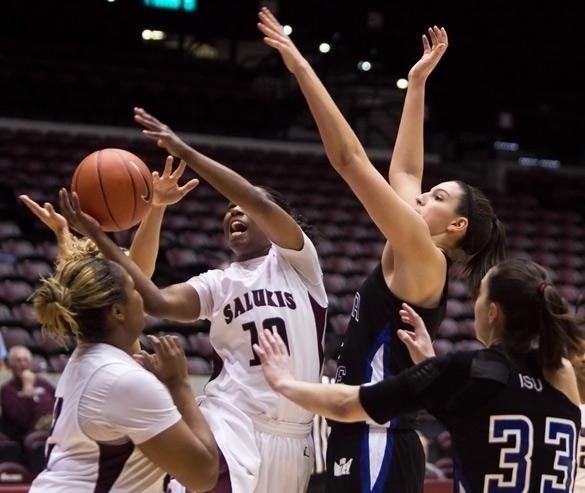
[0,344,57,468]
[18,155,200,280]
[26,256,219,493]
[56,105,329,492]
[253,257,580,491]
[256,5,509,491]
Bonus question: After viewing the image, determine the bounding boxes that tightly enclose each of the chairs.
[0,430,24,467]
[325,155,584,377]
[24,425,53,470]
[0,121,324,377]
[0,455,35,492]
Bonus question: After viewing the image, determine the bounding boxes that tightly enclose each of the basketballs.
[71,148,153,232]
